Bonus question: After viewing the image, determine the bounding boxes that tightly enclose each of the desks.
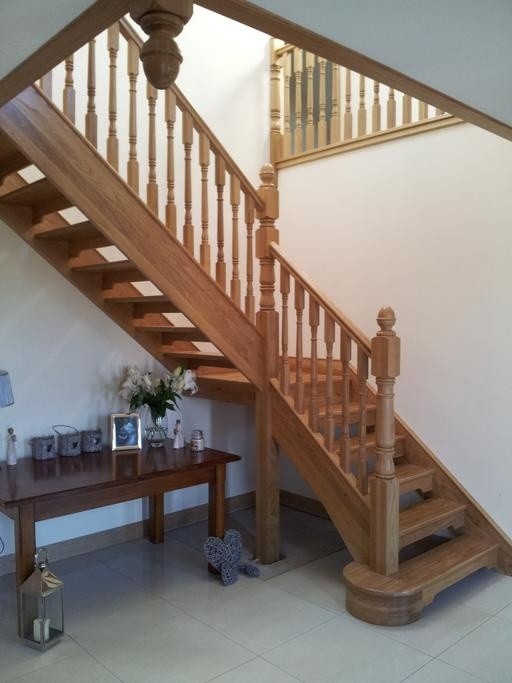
[0,438,241,638]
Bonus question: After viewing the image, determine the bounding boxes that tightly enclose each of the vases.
[144,407,168,448]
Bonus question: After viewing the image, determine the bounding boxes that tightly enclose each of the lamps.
[19,548,64,652]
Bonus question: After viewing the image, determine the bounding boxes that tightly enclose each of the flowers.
[117,364,198,442]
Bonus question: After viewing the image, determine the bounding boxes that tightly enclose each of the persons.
[117,419,136,445]
[6,428,17,465]
[172,420,185,449]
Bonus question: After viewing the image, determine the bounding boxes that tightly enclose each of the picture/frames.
[111,412,141,451]
